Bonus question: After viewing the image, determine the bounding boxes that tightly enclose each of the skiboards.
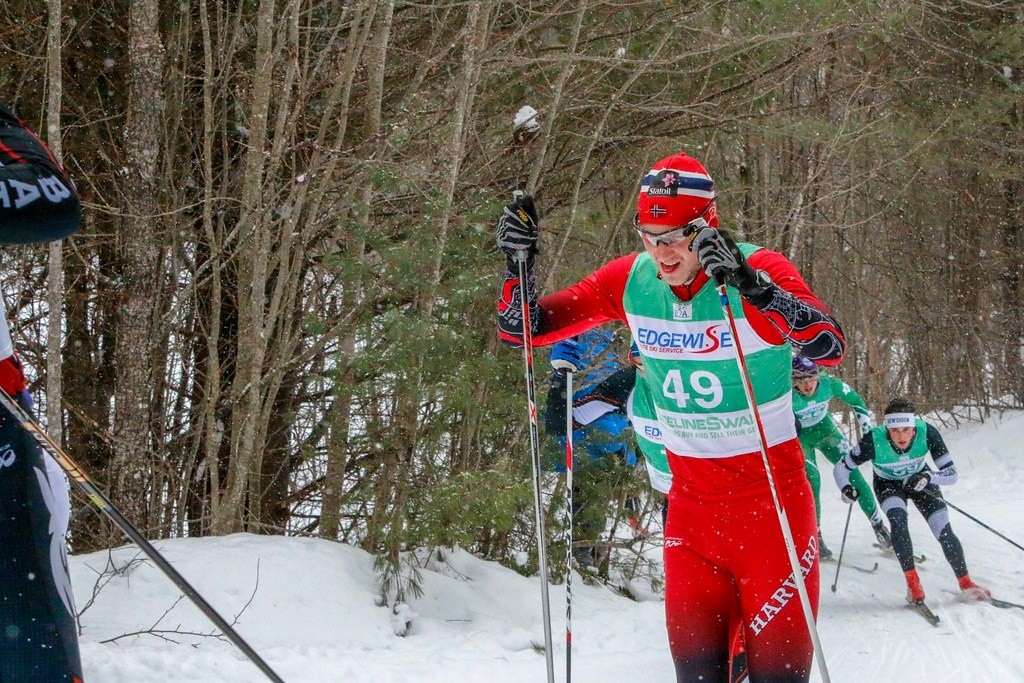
[817,541,926,576]
[904,588,1024,626]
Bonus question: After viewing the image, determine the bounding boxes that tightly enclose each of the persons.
[791,353,898,563]
[0,95,81,683]
[497,152,845,683]
[545,323,653,590]
[833,394,992,601]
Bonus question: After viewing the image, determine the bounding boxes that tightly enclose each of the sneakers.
[818,526,832,560]
[905,568,925,601]
[872,519,892,547]
[957,573,990,601]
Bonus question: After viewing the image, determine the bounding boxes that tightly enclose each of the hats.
[629,333,640,356]
[792,353,819,372]
[638,152,719,228]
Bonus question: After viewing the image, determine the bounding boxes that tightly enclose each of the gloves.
[683,225,774,304]
[496,190,540,273]
[903,473,931,497]
[841,485,860,503]
[550,339,581,379]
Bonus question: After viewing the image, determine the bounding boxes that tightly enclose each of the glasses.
[791,369,819,383]
[635,364,644,371]
[634,194,718,247]
